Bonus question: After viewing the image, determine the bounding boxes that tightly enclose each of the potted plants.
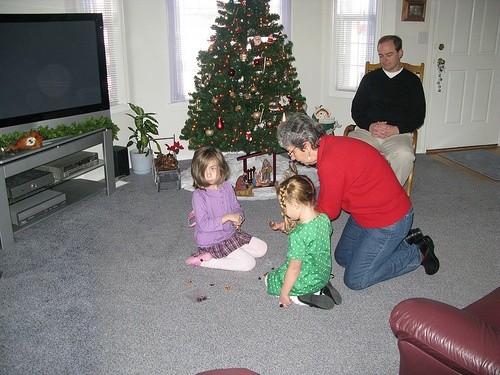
[125,103,158,176]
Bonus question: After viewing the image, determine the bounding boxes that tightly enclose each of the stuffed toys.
[312,108,329,121]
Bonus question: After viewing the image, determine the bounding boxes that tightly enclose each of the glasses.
[287,146,297,157]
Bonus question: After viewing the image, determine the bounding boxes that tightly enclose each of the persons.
[185,147,268,272]
[278,112,440,290]
[348,35,426,188]
[265,175,342,309]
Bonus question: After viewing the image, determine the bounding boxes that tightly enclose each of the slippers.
[184,252,212,267]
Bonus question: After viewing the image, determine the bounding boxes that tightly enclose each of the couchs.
[389,288,500,375]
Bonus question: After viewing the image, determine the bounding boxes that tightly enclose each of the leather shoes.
[413,235,440,276]
[404,228,424,246]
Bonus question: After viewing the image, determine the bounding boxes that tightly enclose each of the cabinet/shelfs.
[0,126,117,250]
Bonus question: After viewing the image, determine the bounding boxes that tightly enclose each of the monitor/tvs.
[0,12,110,128]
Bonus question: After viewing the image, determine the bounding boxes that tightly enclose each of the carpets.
[438,150,500,183]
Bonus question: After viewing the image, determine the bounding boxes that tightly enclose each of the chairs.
[342,61,425,197]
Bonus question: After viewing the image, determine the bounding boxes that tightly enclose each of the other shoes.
[296,292,334,311]
[320,277,343,306]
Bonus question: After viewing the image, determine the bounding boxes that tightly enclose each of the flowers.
[161,141,185,163]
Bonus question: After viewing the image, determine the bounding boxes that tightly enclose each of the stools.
[152,159,181,192]
